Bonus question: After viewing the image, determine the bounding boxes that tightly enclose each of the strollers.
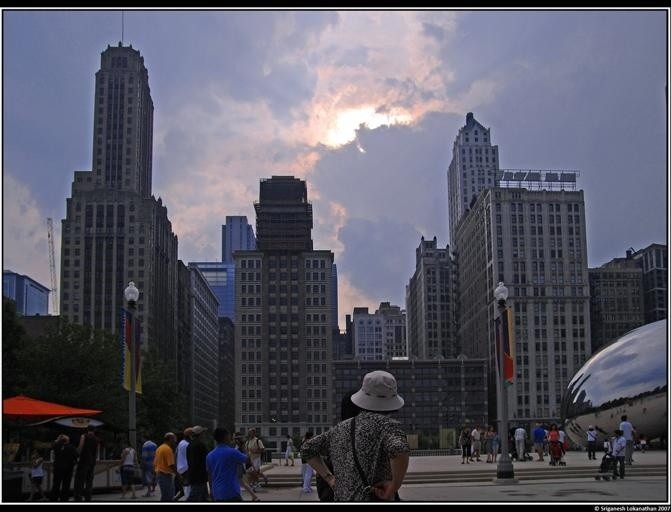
[595,453,618,483]
[509,424,535,461]
[546,440,568,467]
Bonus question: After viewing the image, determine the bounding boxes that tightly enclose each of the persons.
[459,415,637,481]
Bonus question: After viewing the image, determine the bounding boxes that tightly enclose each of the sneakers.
[612,458,634,480]
[588,456,597,460]
[462,458,497,464]
[536,452,549,462]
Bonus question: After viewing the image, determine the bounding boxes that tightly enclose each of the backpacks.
[63,445,79,470]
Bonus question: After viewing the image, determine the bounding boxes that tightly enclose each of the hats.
[184,427,193,436]
[350,370,405,412]
[191,426,209,435]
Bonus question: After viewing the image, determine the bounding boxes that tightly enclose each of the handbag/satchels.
[370,488,401,502]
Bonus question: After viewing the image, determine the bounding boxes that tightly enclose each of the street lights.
[490,278,516,483]
[123,280,144,495]
